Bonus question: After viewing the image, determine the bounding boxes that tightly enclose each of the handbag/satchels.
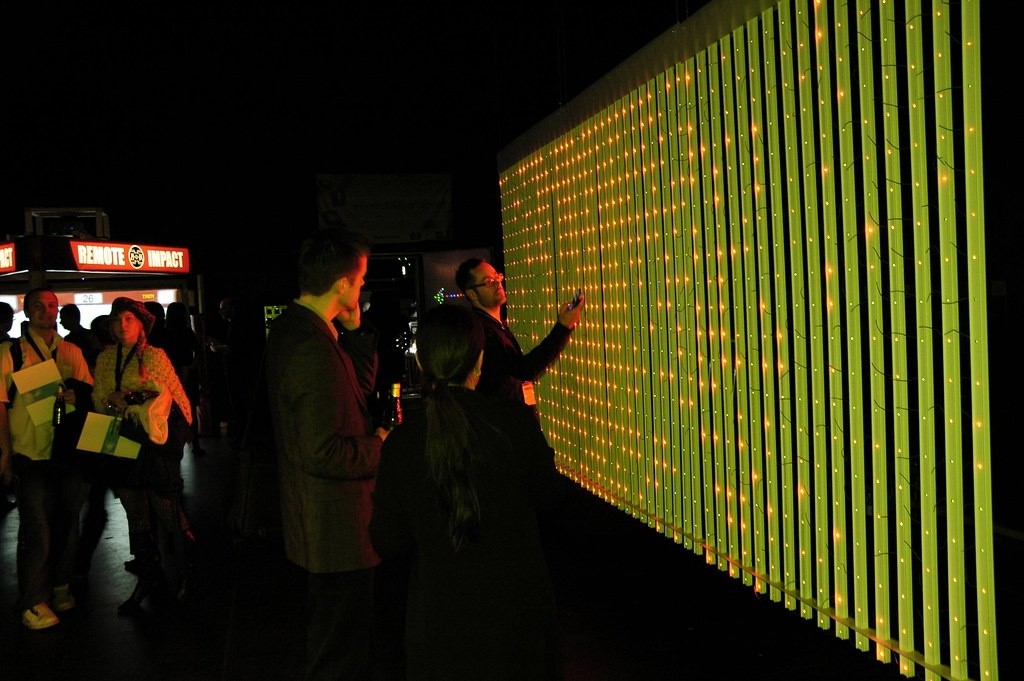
[220,445,285,557]
[555,615,635,681]
[77,408,156,476]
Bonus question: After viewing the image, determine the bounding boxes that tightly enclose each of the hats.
[112,297,156,336]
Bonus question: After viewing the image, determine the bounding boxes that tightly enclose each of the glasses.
[468,273,504,289]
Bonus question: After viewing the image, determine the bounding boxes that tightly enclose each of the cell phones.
[568,289,581,310]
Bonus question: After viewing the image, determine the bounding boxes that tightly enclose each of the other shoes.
[21,601,60,630]
[52,583,76,612]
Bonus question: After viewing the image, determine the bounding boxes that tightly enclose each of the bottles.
[123,389,146,405]
[51,384,67,428]
[101,398,117,417]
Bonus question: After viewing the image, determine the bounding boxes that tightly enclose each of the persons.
[0,302,12,342]
[369,304,571,681]
[377,303,410,426]
[91,296,194,611]
[165,302,206,457]
[60,304,100,368]
[0,287,94,629]
[210,298,246,446]
[263,225,393,681]
[88,315,117,368]
[143,301,165,346]
[456,256,581,404]
